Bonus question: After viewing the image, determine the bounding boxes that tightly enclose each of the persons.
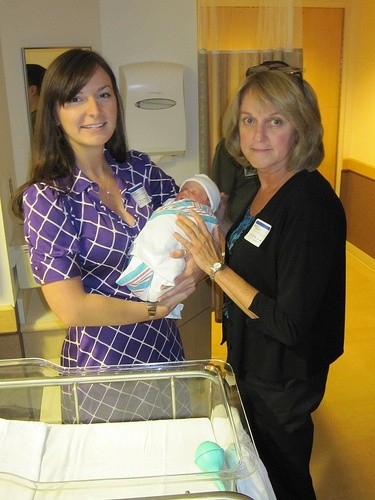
[26,64,48,135]
[116,175,221,303]
[11,48,203,425]
[211,60,347,500]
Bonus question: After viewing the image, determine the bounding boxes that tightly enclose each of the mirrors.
[22,46,93,164]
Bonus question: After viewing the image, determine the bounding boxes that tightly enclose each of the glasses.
[244,64,304,89]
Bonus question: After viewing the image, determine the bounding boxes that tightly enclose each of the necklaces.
[81,165,110,195]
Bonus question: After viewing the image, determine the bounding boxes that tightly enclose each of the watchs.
[146,301,157,321]
[210,261,226,282]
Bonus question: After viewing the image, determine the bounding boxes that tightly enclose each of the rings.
[184,249,187,259]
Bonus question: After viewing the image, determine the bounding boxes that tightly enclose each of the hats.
[179,173,221,214]
